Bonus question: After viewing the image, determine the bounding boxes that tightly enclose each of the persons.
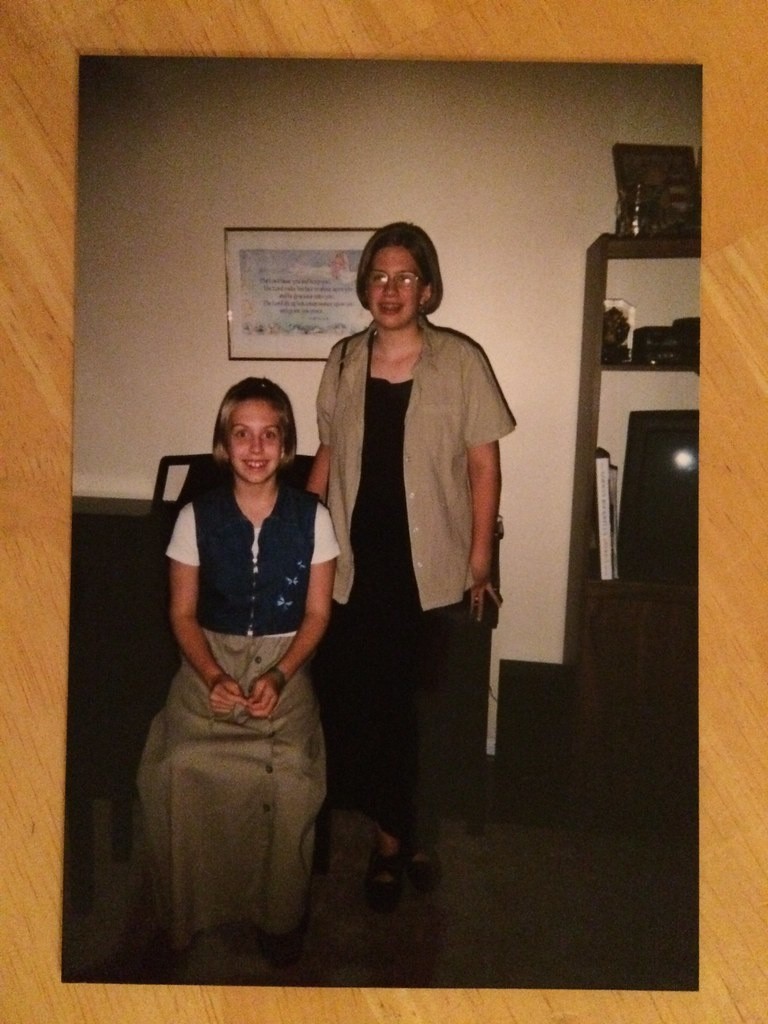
[139,376,341,975]
[307,224,515,906]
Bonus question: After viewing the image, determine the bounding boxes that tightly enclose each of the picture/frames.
[223,225,382,363]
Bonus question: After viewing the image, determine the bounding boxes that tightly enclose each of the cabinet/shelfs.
[558,233,702,789]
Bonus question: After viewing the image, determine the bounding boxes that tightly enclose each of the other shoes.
[169,936,207,979]
[257,925,306,971]
[404,841,442,893]
[362,845,401,913]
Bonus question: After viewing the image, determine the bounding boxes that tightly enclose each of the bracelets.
[270,664,288,687]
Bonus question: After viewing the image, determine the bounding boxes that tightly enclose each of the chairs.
[108,451,332,876]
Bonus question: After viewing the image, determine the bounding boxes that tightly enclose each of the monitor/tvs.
[616,410,698,586]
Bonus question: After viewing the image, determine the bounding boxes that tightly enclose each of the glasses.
[365,269,426,288]
